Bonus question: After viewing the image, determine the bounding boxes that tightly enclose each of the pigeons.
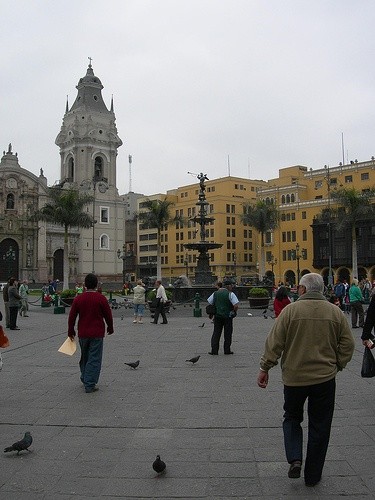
[3,431,34,456]
[124,360,140,370]
[270,315,276,319]
[198,323,205,328]
[185,355,200,365]
[264,315,268,319]
[152,454,166,478]
[262,308,267,314]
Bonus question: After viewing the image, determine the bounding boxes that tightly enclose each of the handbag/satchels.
[0,325,10,348]
[229,310,237,318]
[206,305,216,319]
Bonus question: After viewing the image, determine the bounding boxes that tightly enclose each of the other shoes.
[150,322,157,324]
[305,481,317,487]
[85,386,100,393]
[224,352,233,354]
[208,352,218,355]
[288,461,301,478]
[19,310,22,316]
[133,320,137,323]
[24,316,29,317]
[139,321,143,324]
[160,323,168,324]
[6,326,20,330]
[352,326,359,328]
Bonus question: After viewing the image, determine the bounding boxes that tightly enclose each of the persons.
[268,281,298,304]
[197,173,209,190]
[323,279,375,305]
[361,294,375,347]
[207,280,239,355]
[40,279,102,304]
[0,311,10,370]
[19,280,29,317]
[211,282,223,323]
[142,283,149,309]
[150,280,168,324]
[132,280,149,324]
[257,273,355,488]
[8,279,23,330]
[274,287,291,317]
[3,275,18,328]
[122,280,134,296]
[68,273,114,393]
[349,279,365,328]
[336,281,345,312]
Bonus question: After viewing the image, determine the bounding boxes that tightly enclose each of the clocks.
[99,183,106,193]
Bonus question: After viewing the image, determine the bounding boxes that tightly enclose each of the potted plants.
[60,289,76,307]
[147,288,173,313]
[248,288,271,309]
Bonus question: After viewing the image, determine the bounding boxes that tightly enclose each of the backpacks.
[336,285,344,298]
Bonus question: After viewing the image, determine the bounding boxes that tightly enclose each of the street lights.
[117,243,136,284]
[81,160,109,272]
[291,243,305,286]
[314,165,343,277]
[268,254,277,283]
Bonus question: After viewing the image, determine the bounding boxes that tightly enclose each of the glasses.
[297,285,301,290]
